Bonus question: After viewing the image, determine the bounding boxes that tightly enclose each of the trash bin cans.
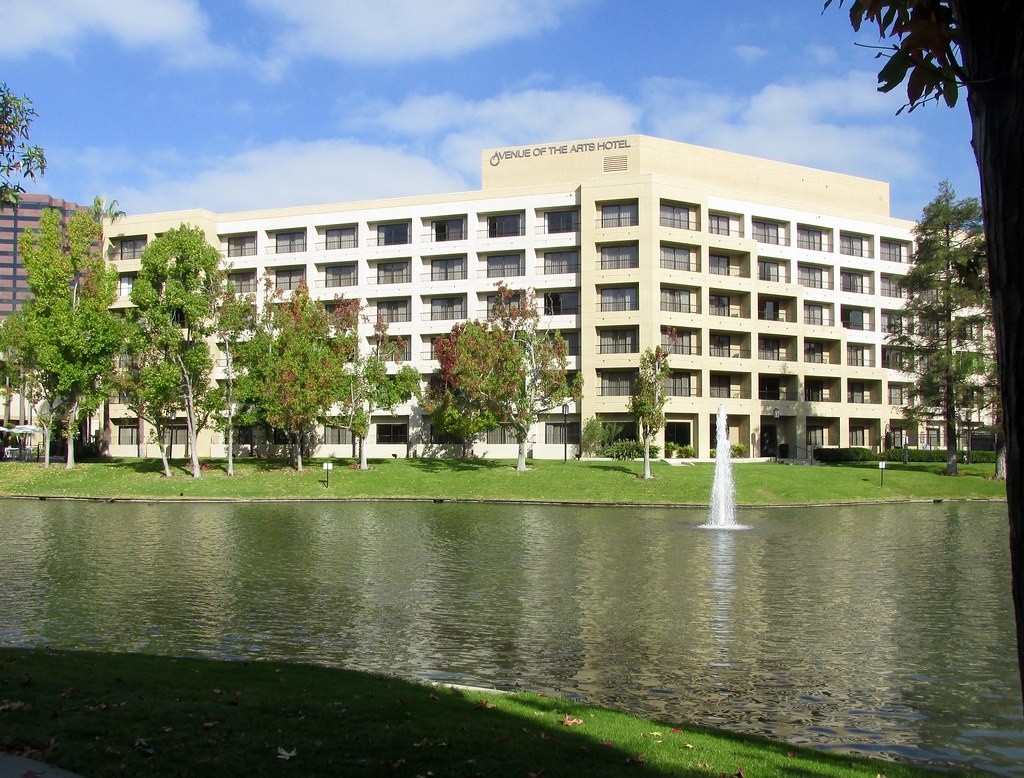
[780,444,789,458]
